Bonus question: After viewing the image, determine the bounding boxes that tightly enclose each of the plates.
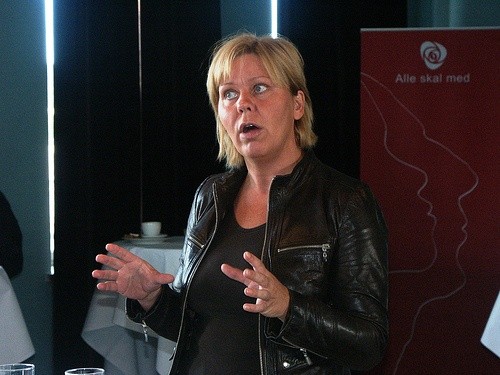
[123,238,167,244]
[142,234,167,238]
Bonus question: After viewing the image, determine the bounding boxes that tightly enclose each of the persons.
[92,33,389,375]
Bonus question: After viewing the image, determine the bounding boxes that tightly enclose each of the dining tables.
[80,237,187,375]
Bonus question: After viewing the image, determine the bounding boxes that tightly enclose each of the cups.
[141,222,161,235]
[0,364,35,375]
[65,368,105,375]
